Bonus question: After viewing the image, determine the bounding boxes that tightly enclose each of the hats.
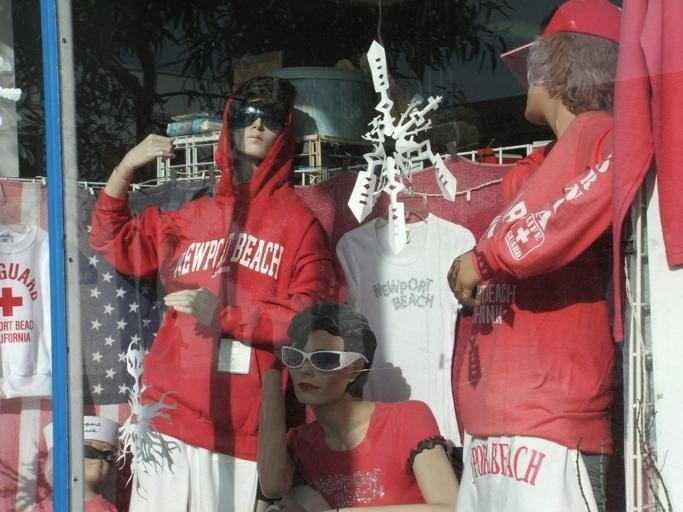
[500,0,622,88]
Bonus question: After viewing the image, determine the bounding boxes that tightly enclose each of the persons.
[447,0,622,512]
[257,303,459,512]
[89,77,328,512]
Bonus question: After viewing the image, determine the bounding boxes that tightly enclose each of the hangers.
[397,141,479,173]
[377,201,427,224]
[0,180,20,225]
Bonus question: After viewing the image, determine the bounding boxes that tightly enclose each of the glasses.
[282,346,369,372]
[231,102,285,135]
